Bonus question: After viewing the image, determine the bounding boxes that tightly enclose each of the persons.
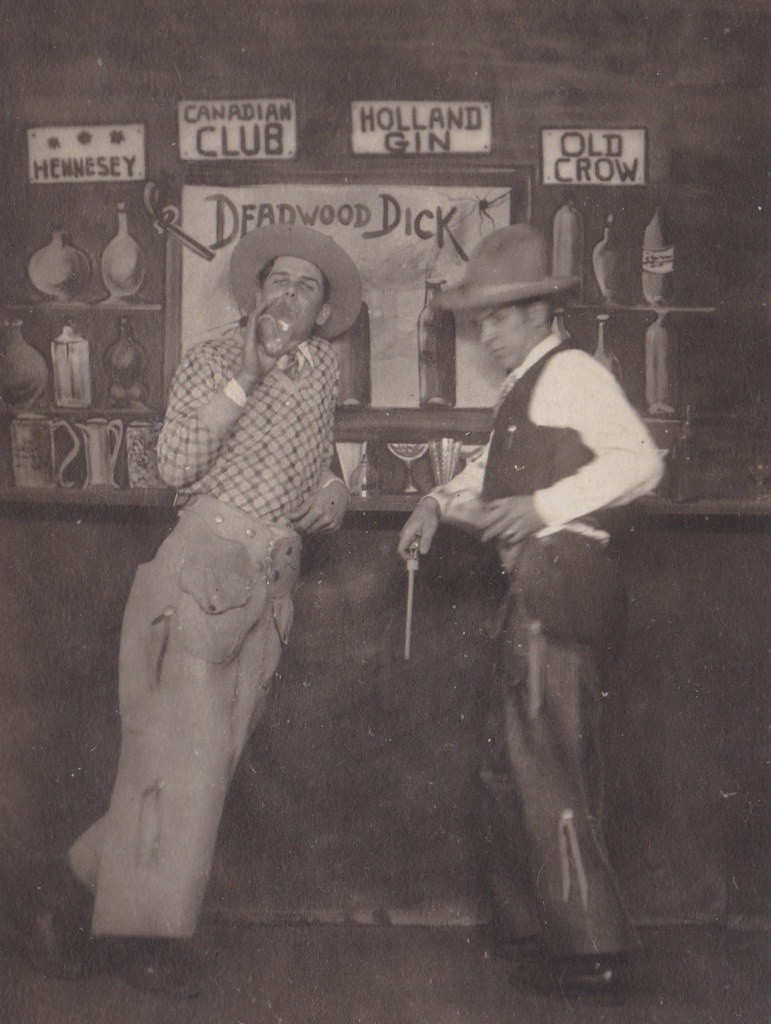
[397,221,668,1006]
[22,225,363,993]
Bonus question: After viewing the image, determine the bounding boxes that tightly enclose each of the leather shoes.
[44,848,94,938]
[492,935,566,961]
[511,951,627,1006]
[99,933,205,999]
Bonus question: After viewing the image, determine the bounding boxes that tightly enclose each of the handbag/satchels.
[416,524,511,600]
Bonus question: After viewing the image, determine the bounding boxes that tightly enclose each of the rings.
[512,523,518,530]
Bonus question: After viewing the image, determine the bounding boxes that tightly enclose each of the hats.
[429,222,581,310]
[229,222,364,340]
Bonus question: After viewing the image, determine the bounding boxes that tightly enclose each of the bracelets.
[422,498,439,512]
